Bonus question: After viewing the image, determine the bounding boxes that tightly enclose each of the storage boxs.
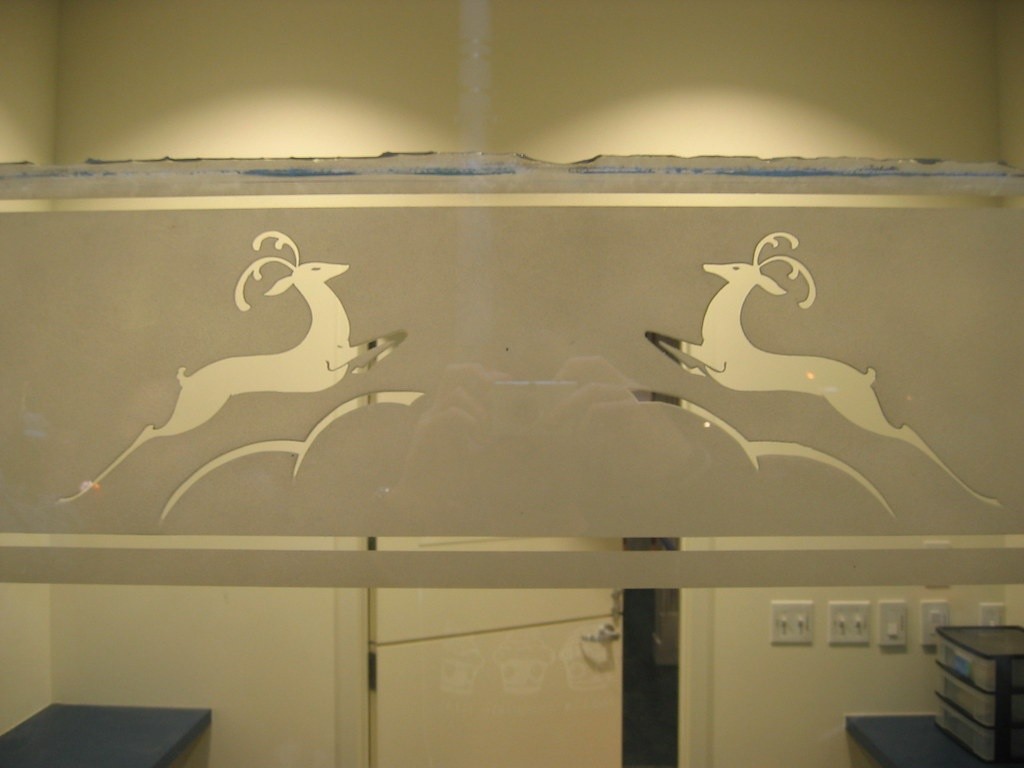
[932,623,1024,754]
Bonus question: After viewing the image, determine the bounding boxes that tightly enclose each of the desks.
[846,715,1024,768]
[0,703,211,768]
